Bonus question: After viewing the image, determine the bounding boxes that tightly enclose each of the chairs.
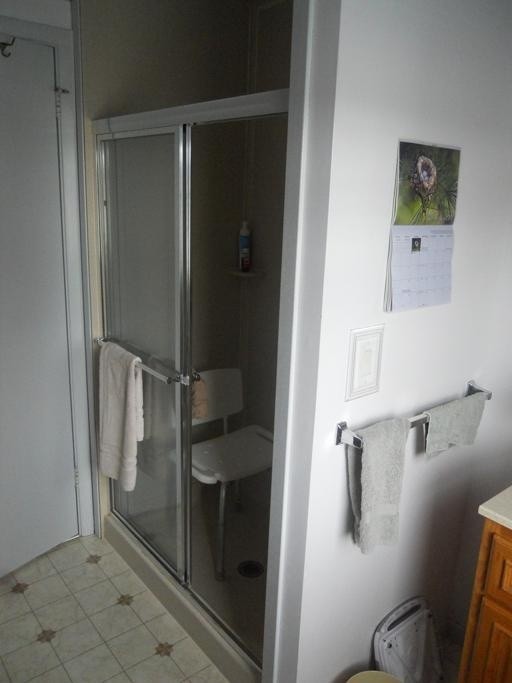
[167,364,271,580]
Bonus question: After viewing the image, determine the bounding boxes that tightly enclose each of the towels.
[423,391,490,457]
[95,340,143,492]
[347,415,403,559]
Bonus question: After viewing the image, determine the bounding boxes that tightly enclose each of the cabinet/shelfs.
[458,518,511,682]
